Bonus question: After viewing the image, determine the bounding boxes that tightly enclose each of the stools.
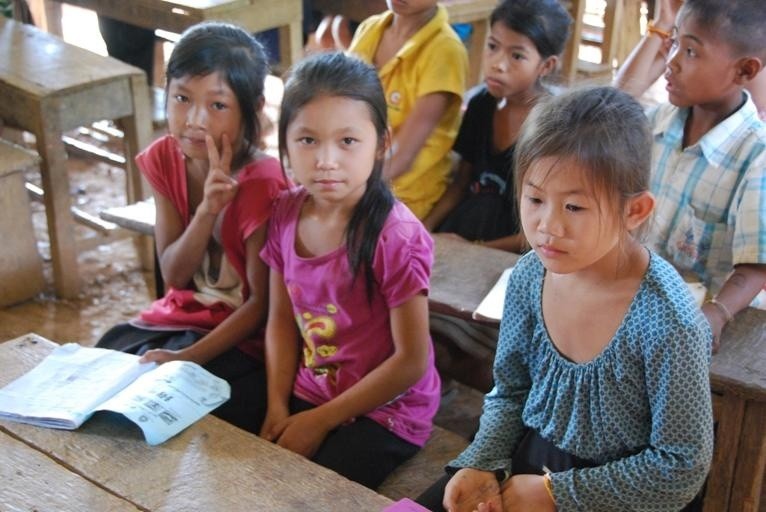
[0,131,45,313]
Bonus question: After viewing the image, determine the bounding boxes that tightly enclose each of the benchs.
[383,424,471,500]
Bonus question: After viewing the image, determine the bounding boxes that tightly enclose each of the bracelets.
[707,298,735,323]
[543,472,560,511]
[646,18,675,38]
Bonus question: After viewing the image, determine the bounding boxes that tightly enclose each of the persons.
[605,0,764,355]
[413,87,714,512]
[227,51,440,490]
[418,0,575,254]
[342,0,469,236]
[90,23,296,433]
[609,0,762,116]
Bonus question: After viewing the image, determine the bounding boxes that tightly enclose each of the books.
[0,342,232,447]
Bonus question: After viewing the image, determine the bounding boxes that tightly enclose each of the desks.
[0,16,161,301]
[560,0,642,87]
[99,185,766,511]
[444,0,502,96]
[39,0,304,118]
[0,331,436,512]
